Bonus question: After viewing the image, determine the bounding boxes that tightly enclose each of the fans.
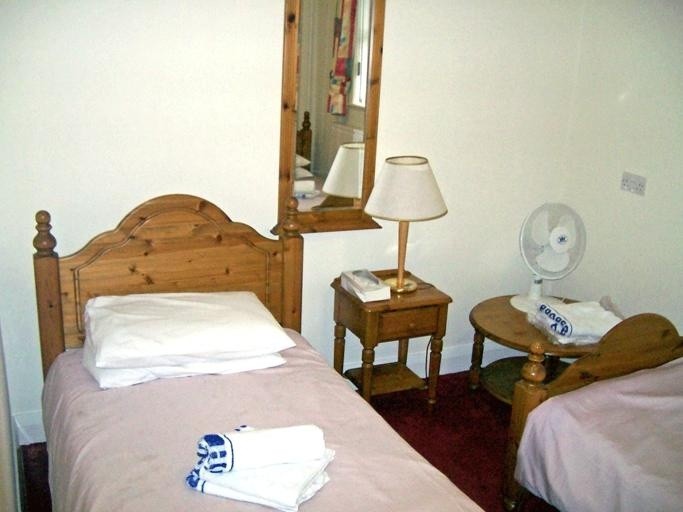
[509,202,587,314]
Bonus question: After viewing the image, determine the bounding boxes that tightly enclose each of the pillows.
[84,289,297,369]
[295,168,313,179]
[294,153,312,167]
[81,337,287,389]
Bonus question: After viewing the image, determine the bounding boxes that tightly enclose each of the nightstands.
[331,270,452,414]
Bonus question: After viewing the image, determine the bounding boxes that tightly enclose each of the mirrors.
[270,0,385,234]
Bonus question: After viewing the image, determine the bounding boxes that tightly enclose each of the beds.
[293,111,328,212]
[33,194,484,512]
[504,314,683,512]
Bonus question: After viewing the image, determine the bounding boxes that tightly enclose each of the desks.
[469,295,597,405]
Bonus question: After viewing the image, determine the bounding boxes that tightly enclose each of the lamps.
[322,142,366,199]
[364,156,448,294]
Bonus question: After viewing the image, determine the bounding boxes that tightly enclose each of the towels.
[186,424,335,512]
[528,299,623,345]
[294,181,321,193]
